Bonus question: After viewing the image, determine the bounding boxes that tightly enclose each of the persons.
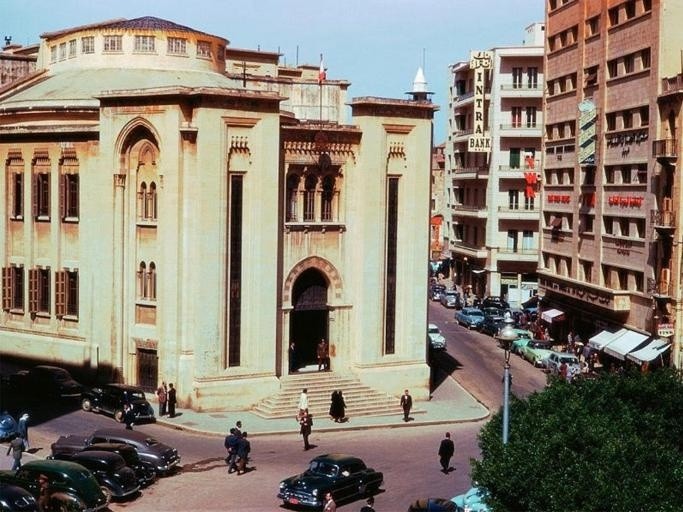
[156,381,168,416]
[399,389,412,422]
[316,337,329,371]
[224,428,239,473]
[322,489,336,511]
[299,408,313,451]
[165,383,177,418]
[437,431,455,474]
[295,387,308,421]
[360,497,375,512]
[516,313,626,383]
[289,342,299,373]
[6,431,25,470]
[118,401,135,430]
[334,391,347,423]
[224,420,243,465]
[16,412,31,452]
[329,390,339,420]
[230,432,250,475]
[501,371,513,392]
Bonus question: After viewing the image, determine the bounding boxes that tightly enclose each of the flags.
[317,56,327,88]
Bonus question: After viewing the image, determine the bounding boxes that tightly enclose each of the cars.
[403,496,468,512]
[80,380,159,427]
[275,452,384,511]
[426,274,583,376]
[7,361,85,401]
[0,424,183,512]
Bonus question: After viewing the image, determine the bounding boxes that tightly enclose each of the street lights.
[490,312,521,442]
